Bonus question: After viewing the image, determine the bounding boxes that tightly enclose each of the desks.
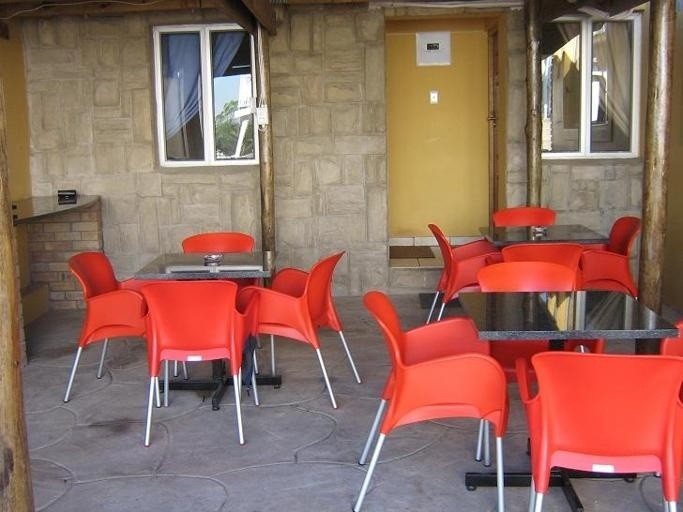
[479,225,610,248]
[11,195,104,310]
[457,291,679,510]
[133,250,283,410]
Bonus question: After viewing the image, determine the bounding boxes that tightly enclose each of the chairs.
[503,243,583,275]
[474,262,579,464]
[182,232,256,253]
[577,216,642,299]
[352,291,509,511]
[511,349,682,511]
[658,322,682,403]
[237,250,362,409]
[428,222,502,322]
[64,251,161,409]
[490,208,556,226]
[129,278,259,446]
